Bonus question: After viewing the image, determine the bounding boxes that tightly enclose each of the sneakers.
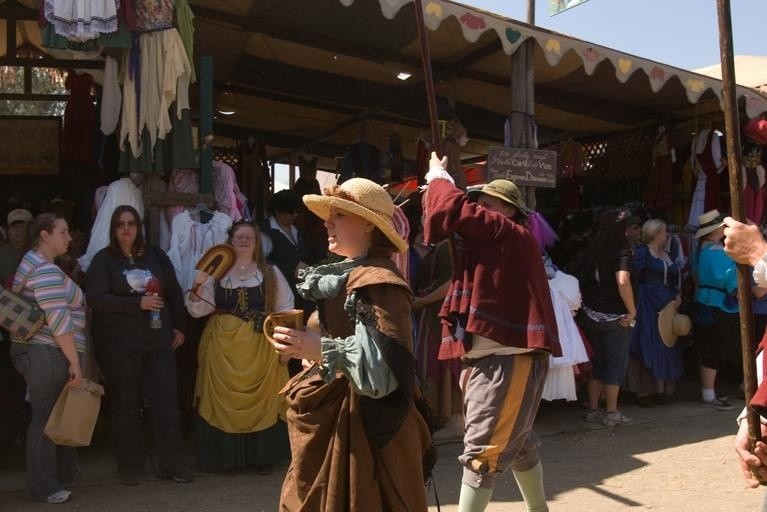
[587,395,655,426]
[45,490,70,503]
[701,394,734,410]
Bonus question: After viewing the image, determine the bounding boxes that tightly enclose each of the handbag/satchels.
[0,289,46,341]
[43,376,105,447]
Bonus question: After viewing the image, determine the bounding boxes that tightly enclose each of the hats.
[302,178,409,252]
[658,301,691,347]
[7,209,33,226]
[467,179,529,223]
[694,209,725,238]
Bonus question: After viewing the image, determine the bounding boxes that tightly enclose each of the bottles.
[150,291,161,329]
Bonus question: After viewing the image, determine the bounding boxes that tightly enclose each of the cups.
[263,308,304,355]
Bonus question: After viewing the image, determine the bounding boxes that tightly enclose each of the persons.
[687,128,725,227]
